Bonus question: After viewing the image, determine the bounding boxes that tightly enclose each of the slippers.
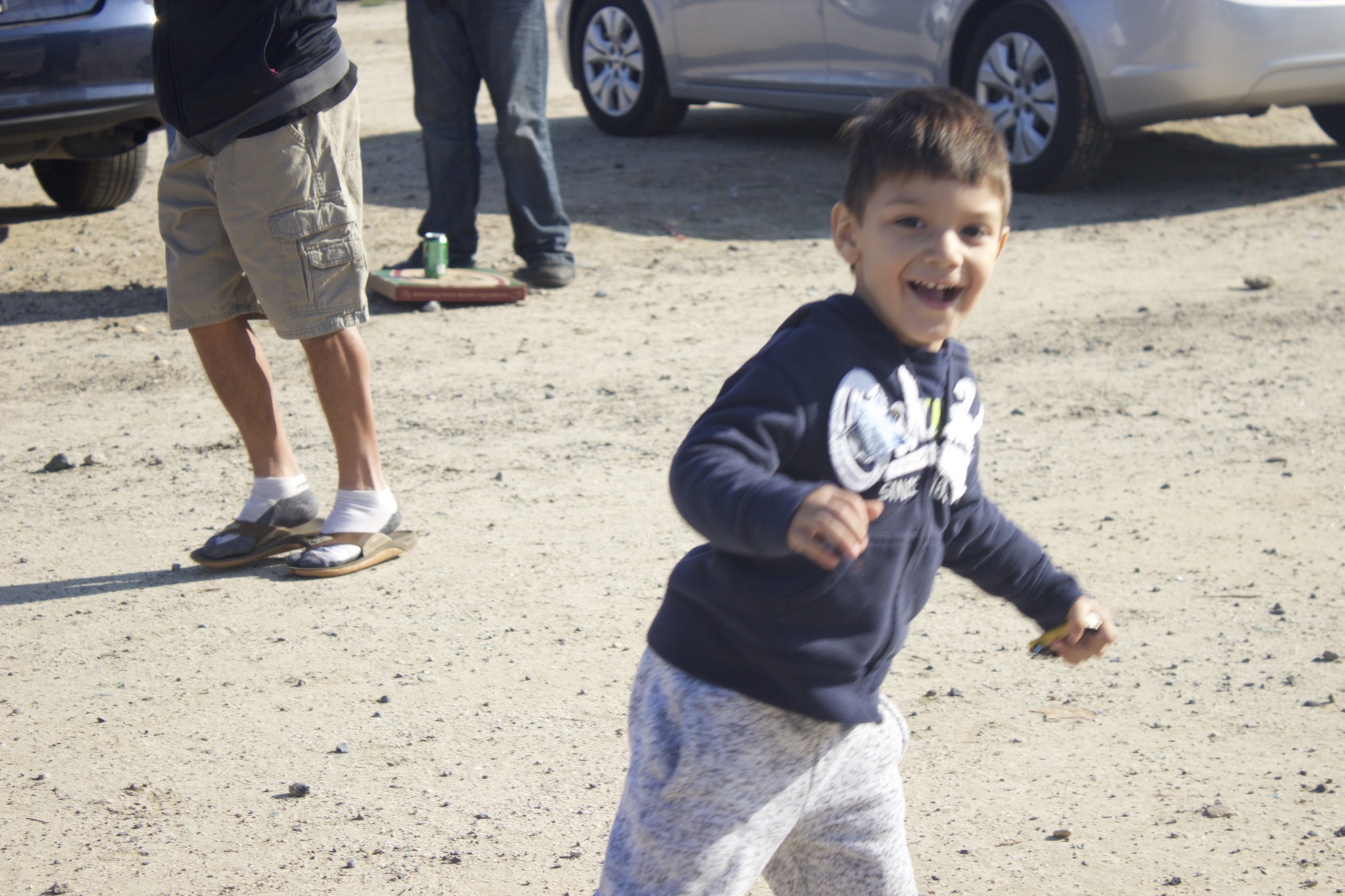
[191,519,326,567]
[286,528,417,577]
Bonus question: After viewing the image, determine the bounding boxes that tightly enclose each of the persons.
[150,1,406,579]
[592,89,1113,896]
[379,1,578,288]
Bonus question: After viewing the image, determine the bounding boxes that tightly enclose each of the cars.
[0,0,165,212]
[554,0,1344,198]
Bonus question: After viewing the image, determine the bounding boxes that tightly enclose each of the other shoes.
[531,262,574,285]
[381,242,477,269]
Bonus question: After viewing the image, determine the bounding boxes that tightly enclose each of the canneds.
[423,232,448,278]
